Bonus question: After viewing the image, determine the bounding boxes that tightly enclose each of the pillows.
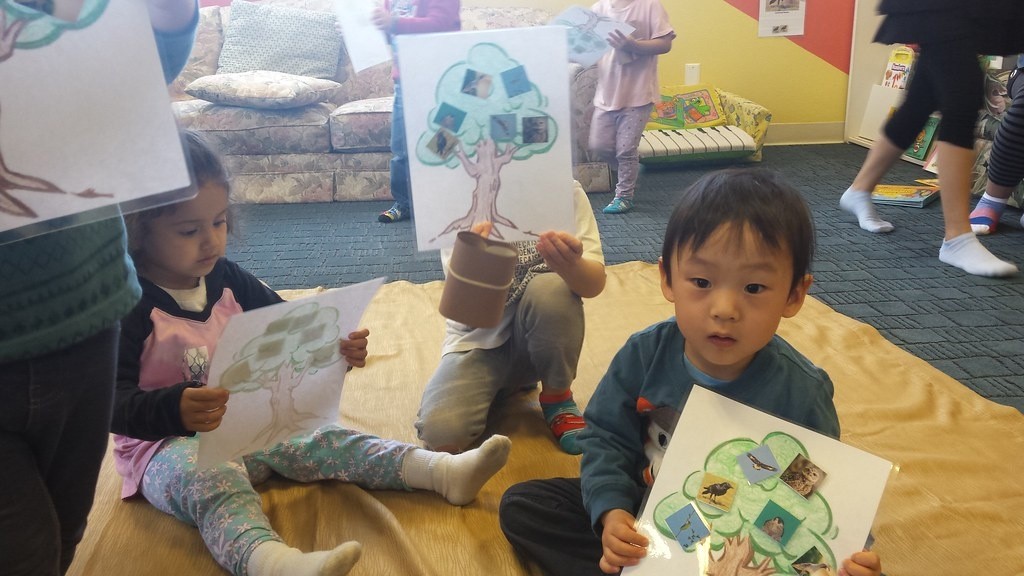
[184,71,342,108]
[217,0,342,80]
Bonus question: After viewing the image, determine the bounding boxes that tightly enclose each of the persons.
[334,1,462,222]
[0,2,202,576]
[108,126,512,576]
[498,164,886,576]
[584,0,678,215]
[839,0,1024,280]
[411,174,608,456]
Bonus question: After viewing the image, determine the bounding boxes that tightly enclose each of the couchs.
[168,0,614,204]
[637,83,771,168]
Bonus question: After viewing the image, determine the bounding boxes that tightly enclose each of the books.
[913,178,942,189]
[870,183,941,209]
[882,43,1004,175]
